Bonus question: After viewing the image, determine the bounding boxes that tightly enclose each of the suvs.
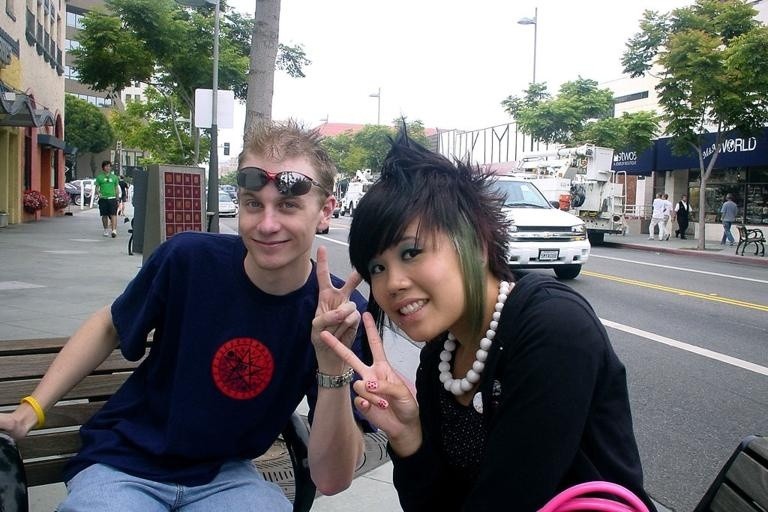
[477,170,596,281]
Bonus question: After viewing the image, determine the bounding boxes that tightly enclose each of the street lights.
[368,88,383,125]
[517,7,539,107]
[176,0,222,217]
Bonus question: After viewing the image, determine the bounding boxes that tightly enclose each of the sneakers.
[103,230,109,236]
[112,230,116,238]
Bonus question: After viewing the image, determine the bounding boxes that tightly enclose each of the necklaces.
[435,277,510,398]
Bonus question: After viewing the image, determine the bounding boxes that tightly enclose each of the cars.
[64,177,98,206]
[332,200,340,218]
[205,184,238,201]
[205,190,237,218]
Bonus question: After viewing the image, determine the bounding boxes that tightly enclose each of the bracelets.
[20,394,45,430]
[118,197,122,204]
[314,367,354,390]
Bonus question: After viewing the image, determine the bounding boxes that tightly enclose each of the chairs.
[735,225,767,257]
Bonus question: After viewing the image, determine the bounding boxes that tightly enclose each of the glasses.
[236,166,330,198]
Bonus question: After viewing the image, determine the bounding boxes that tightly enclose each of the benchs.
[646,433,768,512]
[0,333,316,512]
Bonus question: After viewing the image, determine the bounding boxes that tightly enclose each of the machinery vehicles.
[498,143,631,245]
[339,167,378,219]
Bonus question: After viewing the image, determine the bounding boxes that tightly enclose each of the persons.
[93,160,121,238]
[661,193,673,240]
[674,194,693,240]
[719,192,737,246]
[0,119,370,512]
[317,122,661,512]
[118,174,129,216]
[646,191,665,241]
